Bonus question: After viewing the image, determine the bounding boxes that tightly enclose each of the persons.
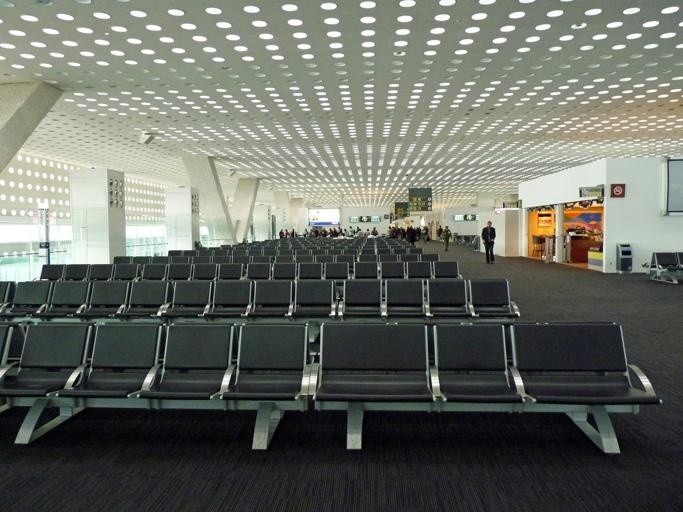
[481,220,497,265]
[278,225,442,246]
[441,226,453,252]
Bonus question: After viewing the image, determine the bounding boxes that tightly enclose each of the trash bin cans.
[616,244,632,274]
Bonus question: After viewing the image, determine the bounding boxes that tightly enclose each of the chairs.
[645,248,681,289]
